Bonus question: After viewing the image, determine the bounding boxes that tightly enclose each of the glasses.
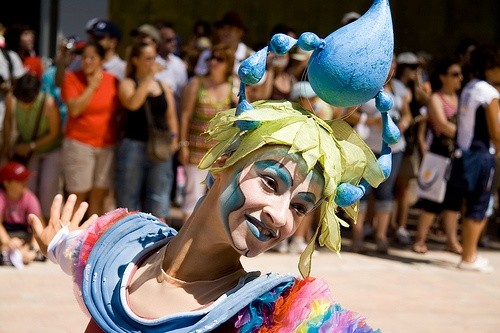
[444,72,463,78]
[210,54,226,63]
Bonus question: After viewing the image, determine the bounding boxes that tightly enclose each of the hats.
[88,20,122,41]
[212,10,250,34]
[396,53,422,66]
[0,162,33,183]
[136,24,162,43]
[342,12,362,26]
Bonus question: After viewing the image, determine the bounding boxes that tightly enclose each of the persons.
[28,96,386,333]
[0,13,500,268]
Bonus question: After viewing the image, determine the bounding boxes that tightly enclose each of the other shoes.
[445,243,466,254]
[414,242,427,252]
[351,240,367,253]
[376,242,388,256]
[395,226,412,245]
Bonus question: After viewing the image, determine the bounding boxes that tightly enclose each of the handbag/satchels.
[415,151,453,203]
[146,130,168,164]
[12,134,33,165]
